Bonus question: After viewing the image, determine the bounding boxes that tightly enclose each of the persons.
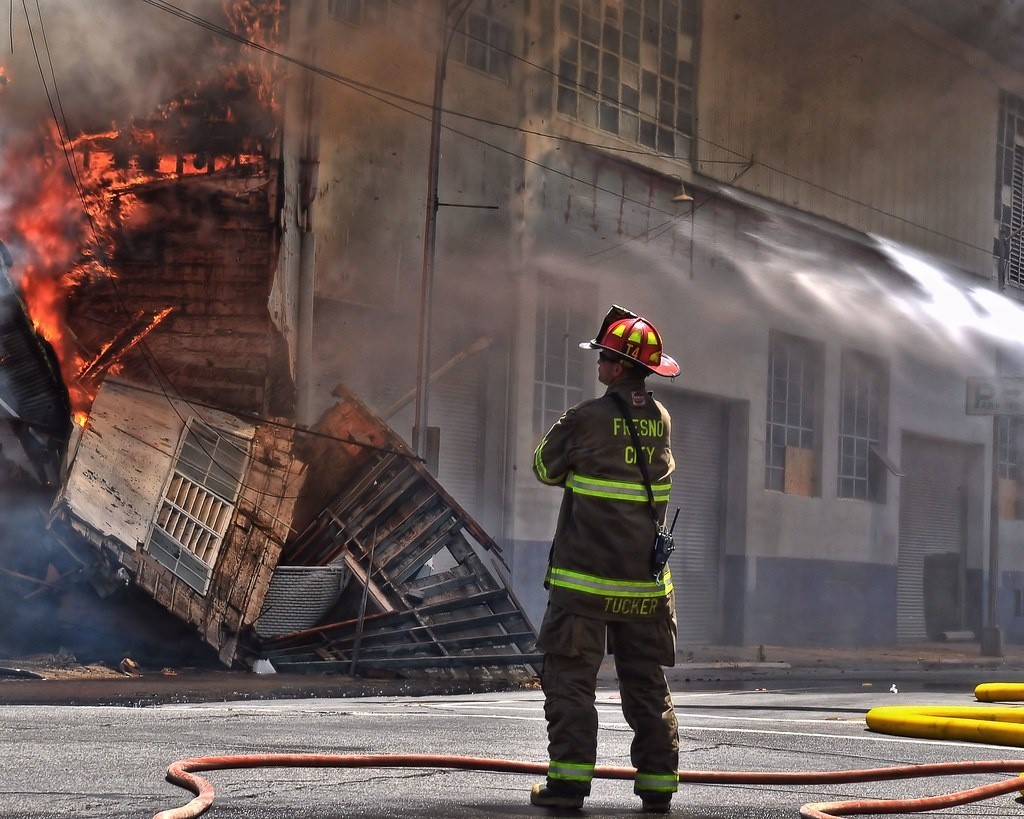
[533,305,679,815]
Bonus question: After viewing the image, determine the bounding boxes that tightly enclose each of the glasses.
[597,351,625,365]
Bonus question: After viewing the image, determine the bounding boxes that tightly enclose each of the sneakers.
[531,782,584,808]
[643,796,671,814]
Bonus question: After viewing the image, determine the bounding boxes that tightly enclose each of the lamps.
[659,172,694,203]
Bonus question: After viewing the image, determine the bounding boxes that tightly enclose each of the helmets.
[579,304,681,383]
